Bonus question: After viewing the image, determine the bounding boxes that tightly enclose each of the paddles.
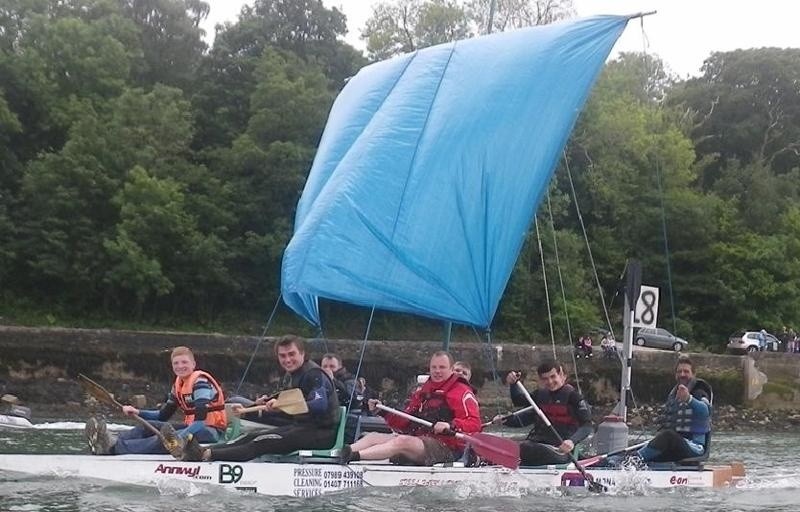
[75,374,161,436]
[515,380,607,493]
[624,262,643,405]
[577,441,647,467]
[376,402,521,469]
[234,388,309,416]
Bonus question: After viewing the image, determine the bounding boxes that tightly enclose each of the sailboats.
[101,6,747,480]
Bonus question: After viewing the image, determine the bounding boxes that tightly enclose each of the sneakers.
[160,421,206,462]
[85,416,119,455]
[341,445,352,465]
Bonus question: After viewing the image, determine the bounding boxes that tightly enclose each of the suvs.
[726,330,784,356]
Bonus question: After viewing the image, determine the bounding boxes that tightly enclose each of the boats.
[0,447,758,505]
[0,403,35,429]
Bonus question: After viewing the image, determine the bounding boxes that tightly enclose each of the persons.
[599,357,713,470]
[85,345,228,455]
[322,332,616,467]
[760,326,800,352]
[160,335,340,462]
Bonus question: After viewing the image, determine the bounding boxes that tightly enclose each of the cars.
[634,325,688,353]
